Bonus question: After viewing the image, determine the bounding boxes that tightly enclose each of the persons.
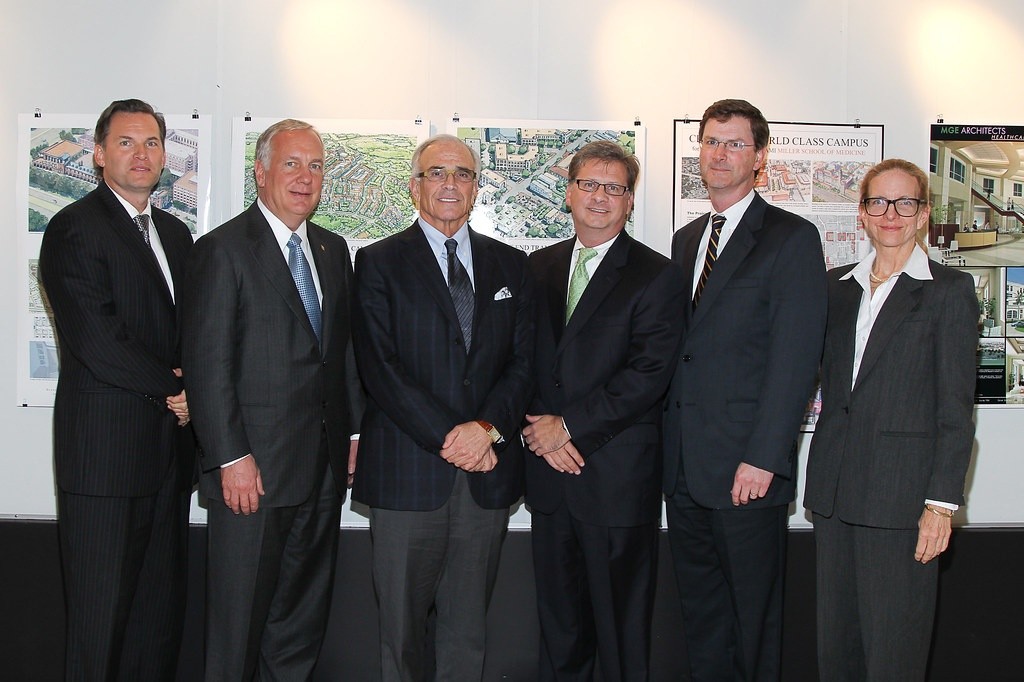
[971,220,978,231]
[803,160,978,682]
[179,115,366,682]
[984,221,990,230]
[350,134,529,682]
[1006,197,1011,210]
[517,141,682,682]
[962,222,970,233]
[994,222,999,241]
[668,99,829,682]
[986,187,992,200]
[1011,200,1015,210]
[39,99,193,682]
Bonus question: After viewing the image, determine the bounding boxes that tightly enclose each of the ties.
[133,214,150,254]
[444,239,475,357]
[693,213,727,314]
[287,232,322,353]
[567,248,598,327]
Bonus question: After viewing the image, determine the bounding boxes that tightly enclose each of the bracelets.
[923,506,954,517]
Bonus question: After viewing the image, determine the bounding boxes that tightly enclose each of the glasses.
[571,179,629,196]
[699,138,756,152]
[861,197,927,217]
[413,167,477,183]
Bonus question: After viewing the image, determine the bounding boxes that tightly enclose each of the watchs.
[477,421,502,443]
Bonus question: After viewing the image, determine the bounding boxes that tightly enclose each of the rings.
[750,493,758,496]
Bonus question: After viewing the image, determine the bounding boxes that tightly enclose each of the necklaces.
[870,272,884,282]
